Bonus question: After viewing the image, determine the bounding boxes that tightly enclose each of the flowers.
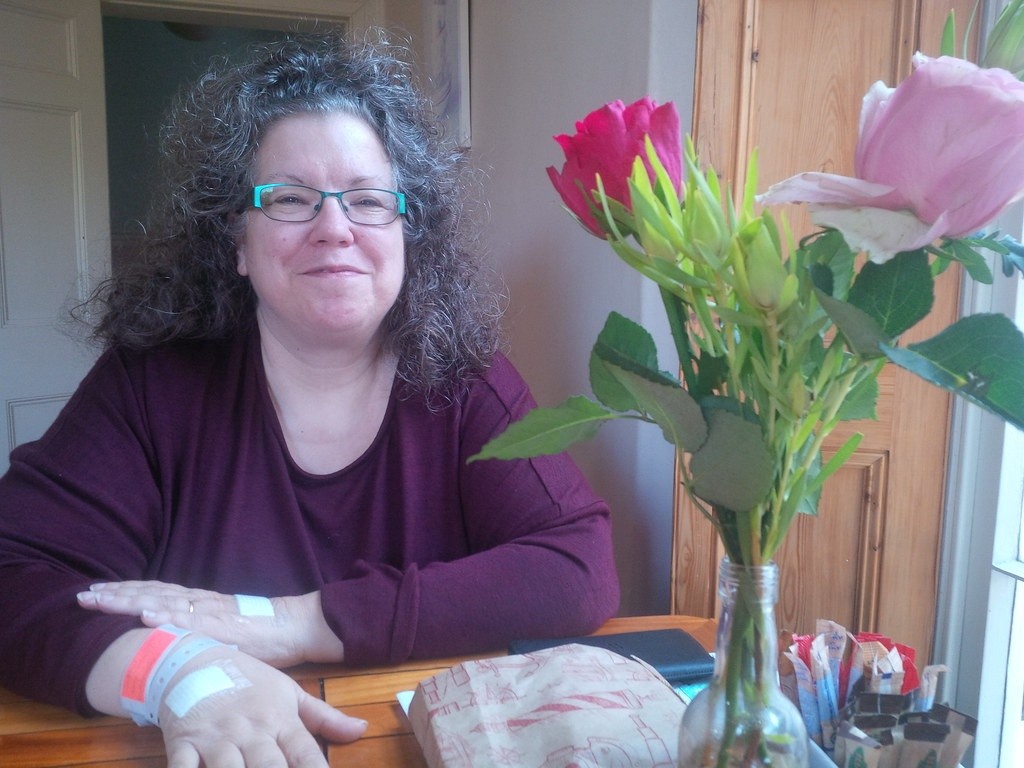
[465,0,1022,562]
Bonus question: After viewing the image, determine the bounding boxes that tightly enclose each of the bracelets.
[145,638,219,728]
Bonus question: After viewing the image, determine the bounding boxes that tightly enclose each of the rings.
[189,601,193,612]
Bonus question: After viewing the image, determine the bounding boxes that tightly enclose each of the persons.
[0,46,622,768]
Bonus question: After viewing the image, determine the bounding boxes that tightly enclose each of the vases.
[676,556,809,768]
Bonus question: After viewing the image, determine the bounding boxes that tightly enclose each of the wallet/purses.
[508,628,715,683]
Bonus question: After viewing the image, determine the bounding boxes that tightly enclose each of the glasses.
[236,181,406,226]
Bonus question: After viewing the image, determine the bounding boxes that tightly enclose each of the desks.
[1,663,326,768]
[318,617,720,766]
